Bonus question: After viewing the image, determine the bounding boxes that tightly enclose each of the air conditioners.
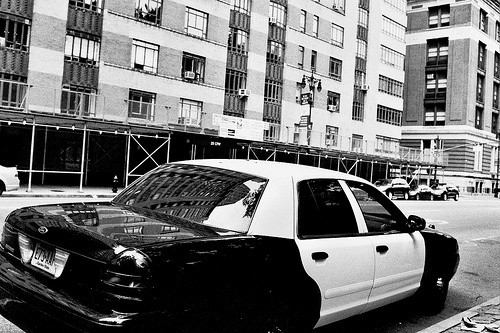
[361,84,370,90]
[269,17,276,24]
[334,5,339,9]
[238,89,250,97]
[328,105,336,111]
[185,71,195,79]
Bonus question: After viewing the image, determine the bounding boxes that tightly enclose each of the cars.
[0,157,462,333]
[0,165,20,196]
[408,185,435,200]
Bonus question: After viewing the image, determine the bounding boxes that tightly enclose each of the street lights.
[301,69,322,165]
[494,128,500,197]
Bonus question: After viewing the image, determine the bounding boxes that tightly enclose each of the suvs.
[374,178,410,200]
[431,183,460,201]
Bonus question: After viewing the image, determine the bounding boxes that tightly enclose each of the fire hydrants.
[112,175,119,192]
